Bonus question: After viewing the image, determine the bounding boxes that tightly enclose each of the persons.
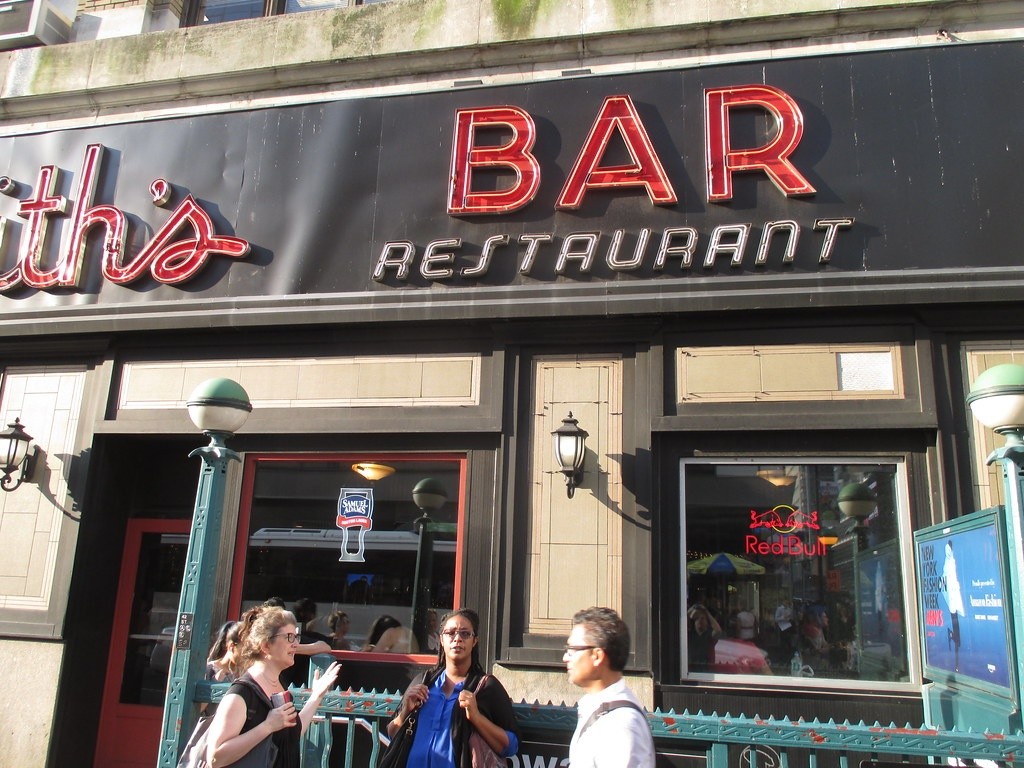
[775,599,855,656]
[385,607,522,768]
[563,607,656,768]
[326,610,352,650]
[688,602,722,671]
[206,605,344,768]
[362,614,420,653]
[425,607,445,655]
[723,612,776,672]
[205,596,333,682]
[291,599,337,650]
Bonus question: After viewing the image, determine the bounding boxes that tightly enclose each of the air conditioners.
[0,0,74,51]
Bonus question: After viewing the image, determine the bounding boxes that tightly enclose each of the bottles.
[791,651,802,677]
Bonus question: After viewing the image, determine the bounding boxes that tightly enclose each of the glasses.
[440,629,477,640]
[562,642,610,658]
[270,629,301,644]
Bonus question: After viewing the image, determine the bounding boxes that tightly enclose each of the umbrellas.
[687,550,766,634]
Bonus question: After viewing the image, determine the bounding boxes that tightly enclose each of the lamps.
[757,463,802,487]
[351,462,396,484]
[0,417,40,492]
[552,410,589,498]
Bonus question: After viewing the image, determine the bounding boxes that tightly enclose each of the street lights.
[408,476,449,654]
[965,363,1023,629]
[836,482,879,680]
[154,376,253,768]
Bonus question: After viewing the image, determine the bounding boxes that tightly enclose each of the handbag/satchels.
[469,674,509,768]
[378,669,429,768]
[176,678,277,768]
